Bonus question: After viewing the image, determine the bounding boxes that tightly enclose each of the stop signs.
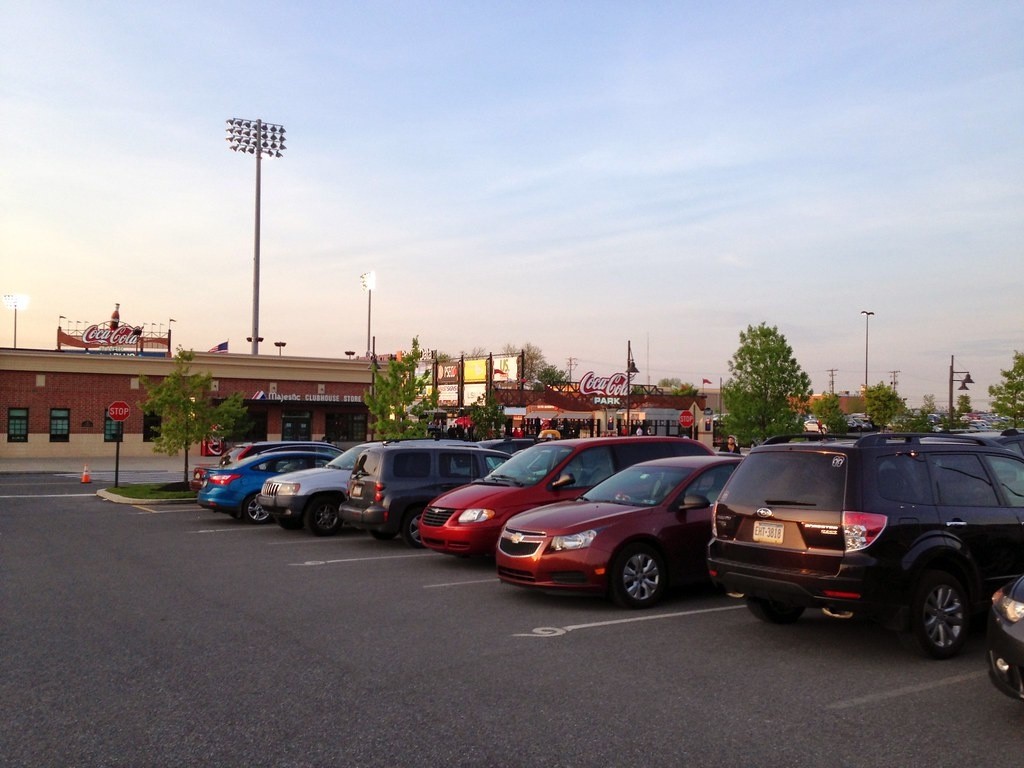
[679,410,694,428]
[108,401,131,422]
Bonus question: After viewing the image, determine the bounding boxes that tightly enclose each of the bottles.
[110,303,120,329]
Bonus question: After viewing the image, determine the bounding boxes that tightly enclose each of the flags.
[208,342,228,353]
[703,379,712,384]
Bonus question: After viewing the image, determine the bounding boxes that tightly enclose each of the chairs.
[526,452,611,486]
[660,473,732,505]
[258,459,304,473]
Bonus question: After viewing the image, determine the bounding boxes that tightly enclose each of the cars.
[254,445,345,458]
[494,456,743,610]
[799,413,1016,433]
[707,413,729,421]
[197,451,335,526]
[218,440,345,468]
[418,434,716,561]
[983,571,1024,708]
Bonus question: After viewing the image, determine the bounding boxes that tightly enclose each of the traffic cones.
[80,464,92,483]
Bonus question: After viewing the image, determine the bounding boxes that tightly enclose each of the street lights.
[5,292,26,348]
[226,119,288,356]
[625,339,639,434]
[861,309,874,389]
[274,341,286,356]
[345,350,356,359]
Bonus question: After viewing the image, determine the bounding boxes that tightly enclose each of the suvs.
[338,438,513,550]
[255,439,395,538]
[706,430,1024,660]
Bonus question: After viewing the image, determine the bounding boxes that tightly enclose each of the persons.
[518,418,594,442]
[427,418,480,446]
[719,435,741,454]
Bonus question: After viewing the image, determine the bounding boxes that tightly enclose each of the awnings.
[556,412,593,419]
[523,411,556,419]
